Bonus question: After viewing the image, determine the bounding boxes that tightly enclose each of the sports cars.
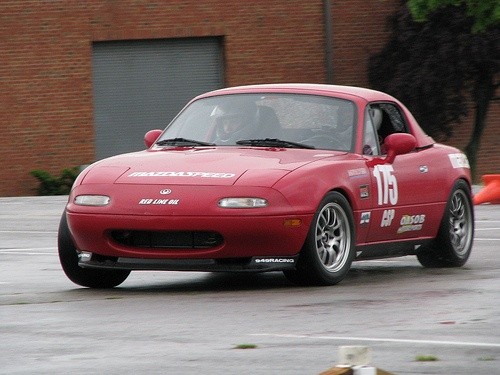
[57,83,475,289]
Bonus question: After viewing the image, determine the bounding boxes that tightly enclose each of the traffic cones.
[471,173,500,205]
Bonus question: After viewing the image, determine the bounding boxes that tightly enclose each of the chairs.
[240,101,283,139]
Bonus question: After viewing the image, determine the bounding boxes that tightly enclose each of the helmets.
[336,103,383,140]
[214,99,260,144]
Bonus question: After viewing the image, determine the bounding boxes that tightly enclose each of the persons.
[324,104,377,155]
[214,100,259,145]
